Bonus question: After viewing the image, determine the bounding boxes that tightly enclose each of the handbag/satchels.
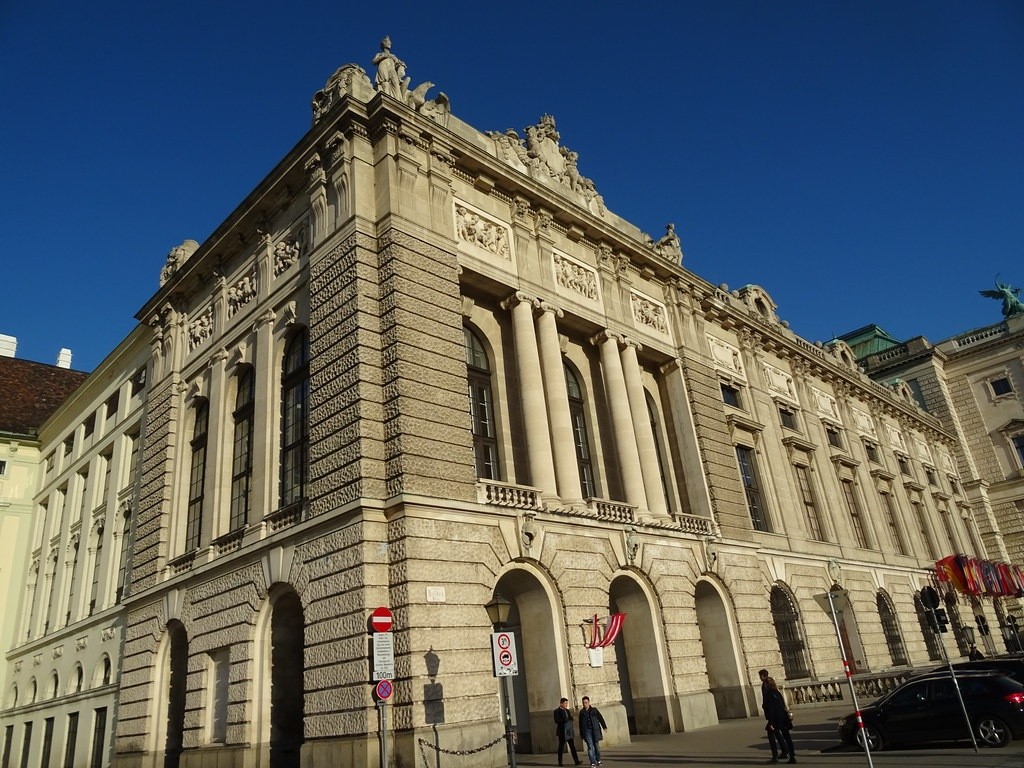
[565,719,575,740]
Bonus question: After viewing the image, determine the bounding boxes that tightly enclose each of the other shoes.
[558,762,562,766]
[779,751,787,758]
[598,761,602,765]
[786,756,797,763]
[767,757,778,765]
[591,763,596,767]
[575,759,582,765]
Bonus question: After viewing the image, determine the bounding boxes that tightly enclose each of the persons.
[995,273,1020,319]
[273,218,309,276]
[227,265,257,319]
[372,34,403,103]
[579,696,608,768]
[969,647,986,661]
[665,223,683,266]
[758,669,798,764]
[554,254,596,299]
[189,306,214,350]
[554,697,584,767]
[631,294,665,333]
[456,206,510,260]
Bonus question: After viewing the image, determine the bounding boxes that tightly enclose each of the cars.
[837,668,1024,752]
[926,656,1024,683]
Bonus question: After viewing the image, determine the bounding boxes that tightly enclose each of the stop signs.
[371,607,393,632]
